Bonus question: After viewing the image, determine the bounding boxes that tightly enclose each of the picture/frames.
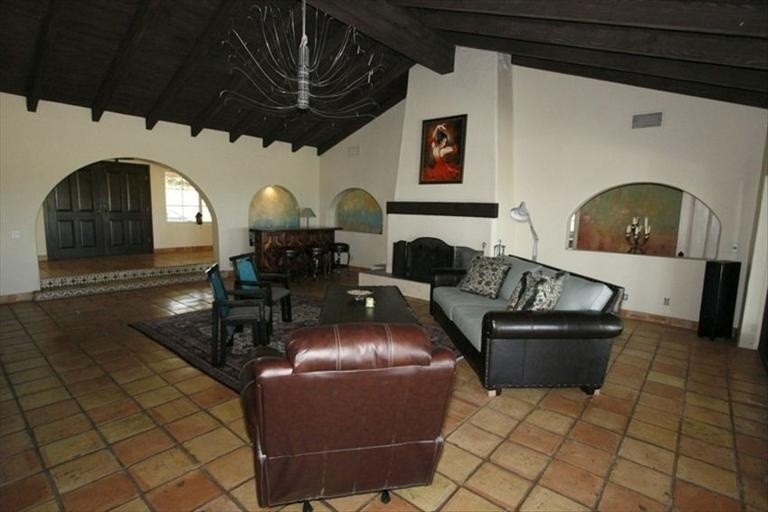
[419,113,467,183]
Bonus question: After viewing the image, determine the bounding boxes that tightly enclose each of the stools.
[328,242,351,271]
[305,244,331,282]
[280,246,303,283]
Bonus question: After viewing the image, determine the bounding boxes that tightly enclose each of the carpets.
[127,289,462,395]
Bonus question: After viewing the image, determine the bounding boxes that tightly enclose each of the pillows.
[461,256,509,299]
[508,271,564,312]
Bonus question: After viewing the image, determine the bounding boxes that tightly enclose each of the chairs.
[206,263,267,366]
[229,253,291,335]
[239,324,456,508]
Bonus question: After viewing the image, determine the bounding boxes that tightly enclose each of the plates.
[347,289,372,301]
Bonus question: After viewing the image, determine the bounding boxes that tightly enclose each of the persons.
[426,123,458,181]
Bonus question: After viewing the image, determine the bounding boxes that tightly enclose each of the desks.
[249,227,344,279]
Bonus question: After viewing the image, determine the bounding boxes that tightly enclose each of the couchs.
[431,253,625,397]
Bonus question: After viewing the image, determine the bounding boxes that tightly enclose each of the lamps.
[218,1,385,118]
[510,202,538,262]
[300,208,317,227]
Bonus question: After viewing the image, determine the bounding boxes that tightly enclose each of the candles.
[625,216,651,239]
[366,298,374,308]
